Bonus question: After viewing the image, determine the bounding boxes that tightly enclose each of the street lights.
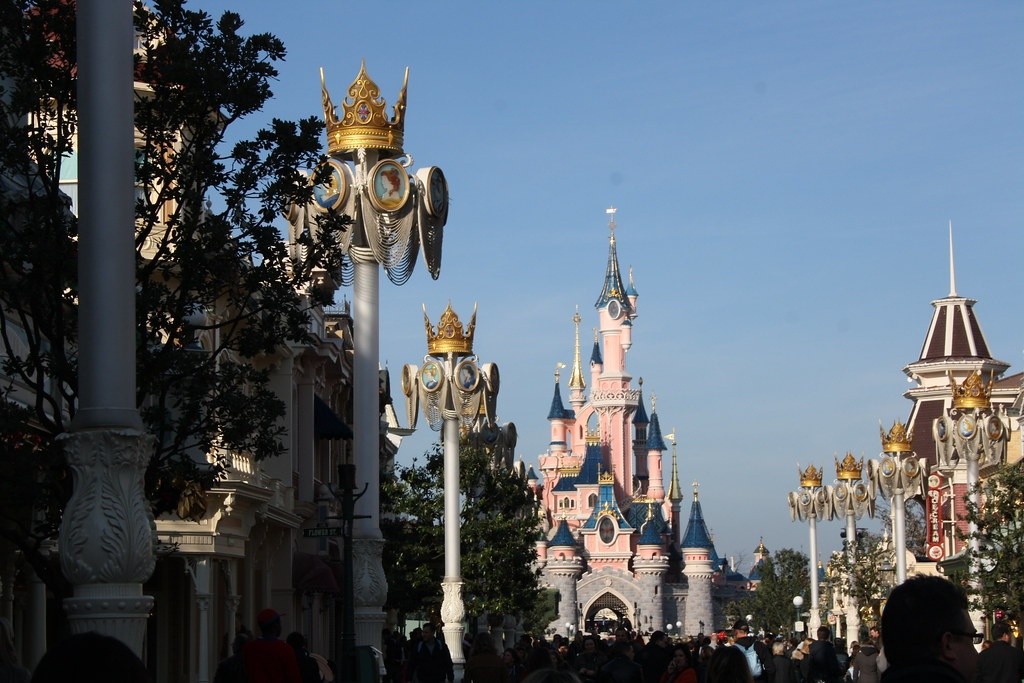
[792,594,804,644]
[867,418,932,587]
[676,620,682,638]
[280,58,453,683]
[936,367,1013,656]
[827,449,878,681]
[565,622,571,644]
[787,460,826,642]
[401,299,501,683]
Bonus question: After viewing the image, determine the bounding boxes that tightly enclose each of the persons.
[383,622,454,683]
[462,616,887,683]
[977,622,1024,683]
[0,616,32,683]
[30,631,152,683]
[214,608,321,683]
[880,575,978,683]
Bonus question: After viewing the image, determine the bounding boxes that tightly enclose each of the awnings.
[294,551,337,594]
